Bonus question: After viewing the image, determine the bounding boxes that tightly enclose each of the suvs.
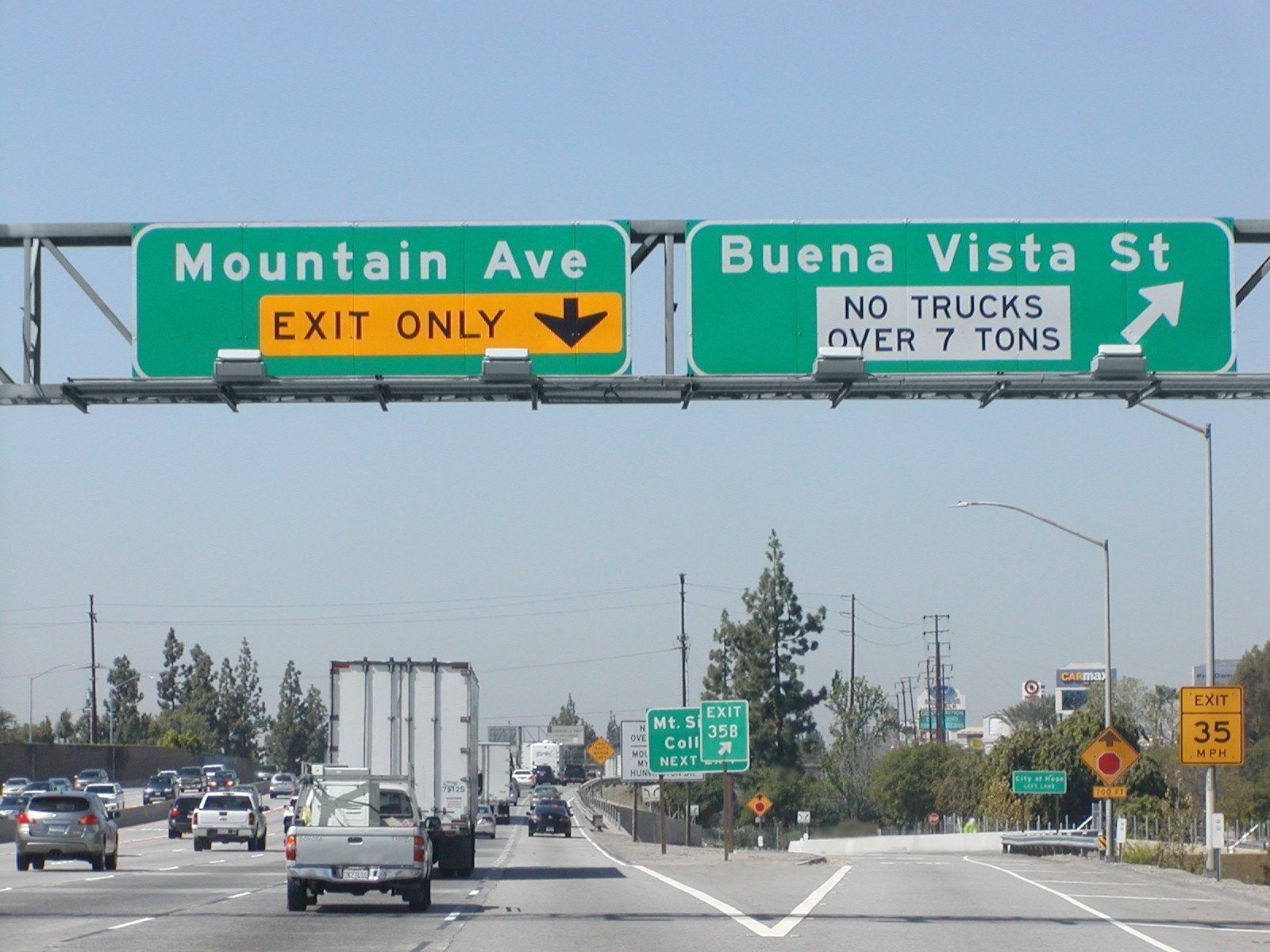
[72,768,109,790]
[81,781,125,810]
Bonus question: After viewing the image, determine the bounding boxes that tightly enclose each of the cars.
[167,794,204,839]
[268,773,296,800]
[0,777,74,819]
[230,785,259,802]
[13,790,120,872]
[474,801,500,840]
[141,763,241,806]
[509,763,606,838]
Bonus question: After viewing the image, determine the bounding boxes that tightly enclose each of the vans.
[253,763,281,786]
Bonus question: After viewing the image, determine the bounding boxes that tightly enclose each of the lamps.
[479,346,532,380]
[214,349,264,382]
[1090,344,1148,377]
[812,346,864,376]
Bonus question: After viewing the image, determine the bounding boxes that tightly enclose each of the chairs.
[383,804,401,815]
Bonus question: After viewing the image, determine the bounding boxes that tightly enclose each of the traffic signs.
[677,217,1243,386]
[127,220,630,382]
[697,699,750,763]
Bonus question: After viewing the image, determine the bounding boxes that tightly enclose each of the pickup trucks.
[285,762,442,910]
[191,791,271,852]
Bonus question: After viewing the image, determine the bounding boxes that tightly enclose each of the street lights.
[28,662,77,742]
[950,501,1119,858]
[108,675,157,743]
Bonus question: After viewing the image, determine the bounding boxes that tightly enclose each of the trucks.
[530,740,567,785]
[478,740,510,826]
[322,655,483,873]
